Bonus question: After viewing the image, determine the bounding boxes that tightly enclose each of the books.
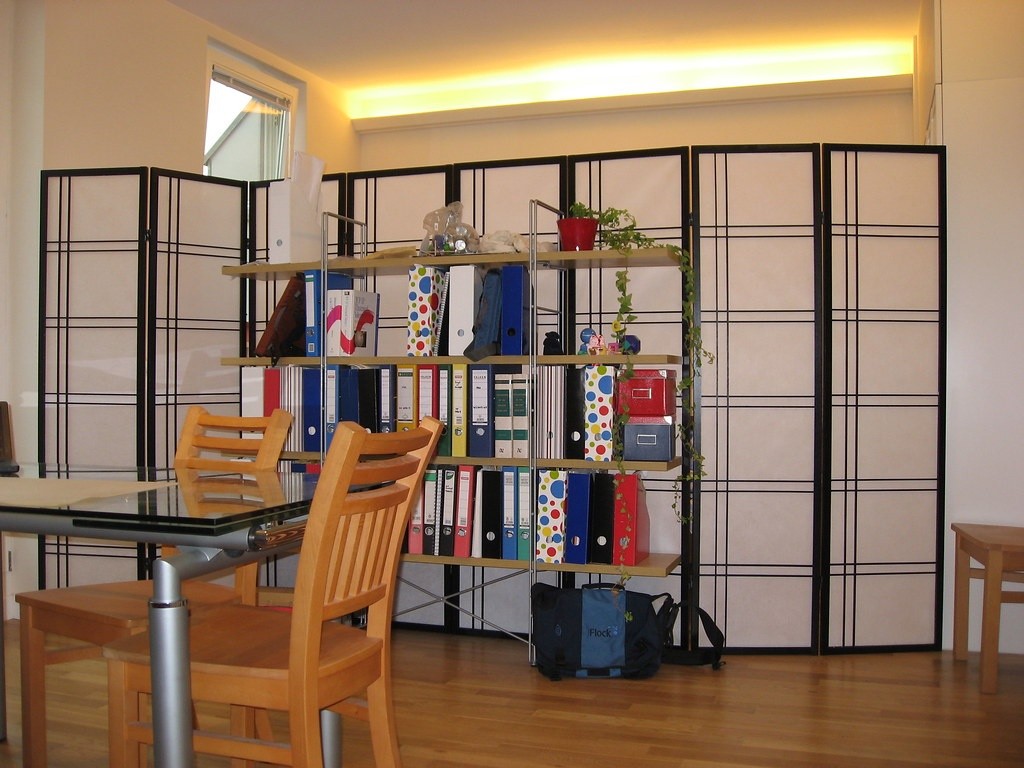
[327,289,379,357]
[435,470,444,556]
[274,367,320,504]
[534,364,581,458]
[433,271,450,356]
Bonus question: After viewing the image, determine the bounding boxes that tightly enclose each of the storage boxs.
[616,368,677,417]
[622,414,677,462]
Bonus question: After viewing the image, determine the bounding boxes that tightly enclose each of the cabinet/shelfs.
[219,248,686,668]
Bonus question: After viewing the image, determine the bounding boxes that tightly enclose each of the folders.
[303,270,354,358]
[302,363,529,460]
[406,464,639,567]
[406,266,445,358]
[582,364,617,465]
[500,264,531,356]
[448,264,485,357]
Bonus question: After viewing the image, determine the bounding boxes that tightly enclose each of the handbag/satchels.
[530,582,723,679]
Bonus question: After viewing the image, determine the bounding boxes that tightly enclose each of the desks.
[0,462,385,767]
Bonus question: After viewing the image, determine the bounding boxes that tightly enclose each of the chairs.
[15,406,294,768]
[951,523,1024,696]
[101,416,446,768]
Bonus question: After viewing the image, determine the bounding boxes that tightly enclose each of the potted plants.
[556,202,601,251]
